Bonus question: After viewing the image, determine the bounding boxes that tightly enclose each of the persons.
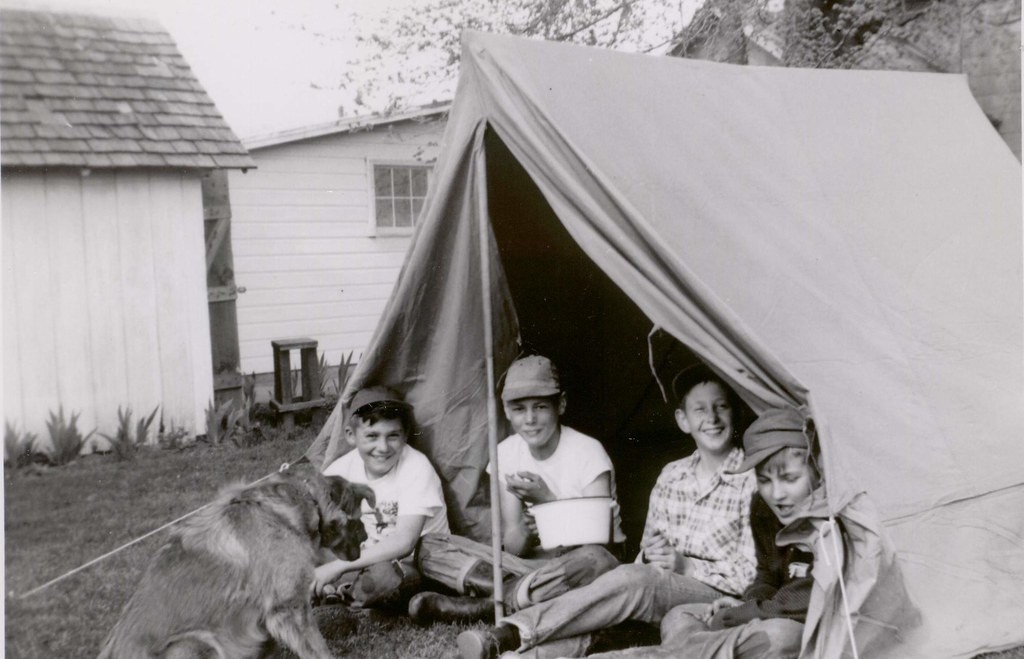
[458,365,821,659]
[310,386,451,608]
[409,356,627,626]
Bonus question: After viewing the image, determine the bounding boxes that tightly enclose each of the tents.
[296,26,1023,659]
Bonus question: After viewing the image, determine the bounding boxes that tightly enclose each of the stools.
[270,337,326,438]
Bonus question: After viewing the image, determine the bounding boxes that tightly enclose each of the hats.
[501,355,561,402]
[347,385,415,422]
[724,409,810,475]
[671,361,722,402]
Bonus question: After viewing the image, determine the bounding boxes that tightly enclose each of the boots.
[408,569,539,628]
[457,555,515,598]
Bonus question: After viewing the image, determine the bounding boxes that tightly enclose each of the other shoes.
[313,604,385,637]
[455,627,500,659]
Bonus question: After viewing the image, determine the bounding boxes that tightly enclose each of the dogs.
[96,463,376,659]
